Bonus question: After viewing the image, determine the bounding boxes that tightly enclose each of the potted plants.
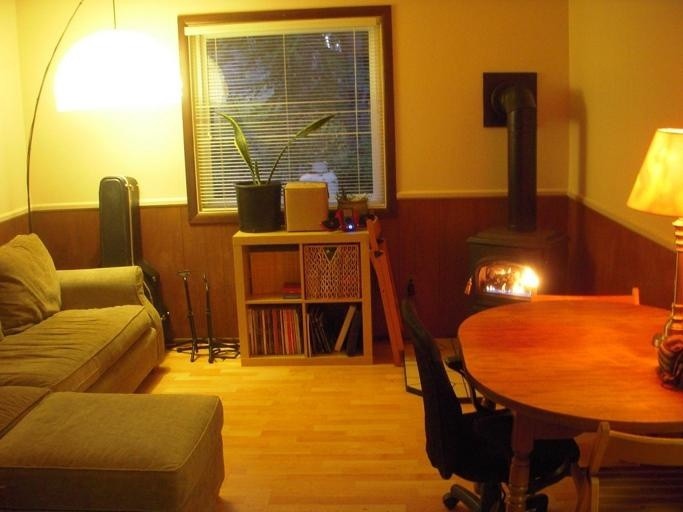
[214,112,334,233]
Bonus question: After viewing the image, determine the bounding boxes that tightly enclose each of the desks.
[457,300,683,512]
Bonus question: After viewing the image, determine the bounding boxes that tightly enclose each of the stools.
[0,392,225,512]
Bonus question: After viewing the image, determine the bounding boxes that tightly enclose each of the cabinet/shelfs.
[231,226,373,365]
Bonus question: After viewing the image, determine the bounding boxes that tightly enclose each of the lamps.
[626,128,683,391]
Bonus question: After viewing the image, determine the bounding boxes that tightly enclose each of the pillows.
[0,233,62,335]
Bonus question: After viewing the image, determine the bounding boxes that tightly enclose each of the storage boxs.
[283,181,330,232]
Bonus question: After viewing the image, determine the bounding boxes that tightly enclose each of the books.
[247,304,362,357]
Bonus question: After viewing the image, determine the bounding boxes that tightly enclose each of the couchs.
[0,265,165,448]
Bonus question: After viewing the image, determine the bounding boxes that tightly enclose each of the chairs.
[570,420,683,512]
[515,286,641,306]
[398,297,581,512]
